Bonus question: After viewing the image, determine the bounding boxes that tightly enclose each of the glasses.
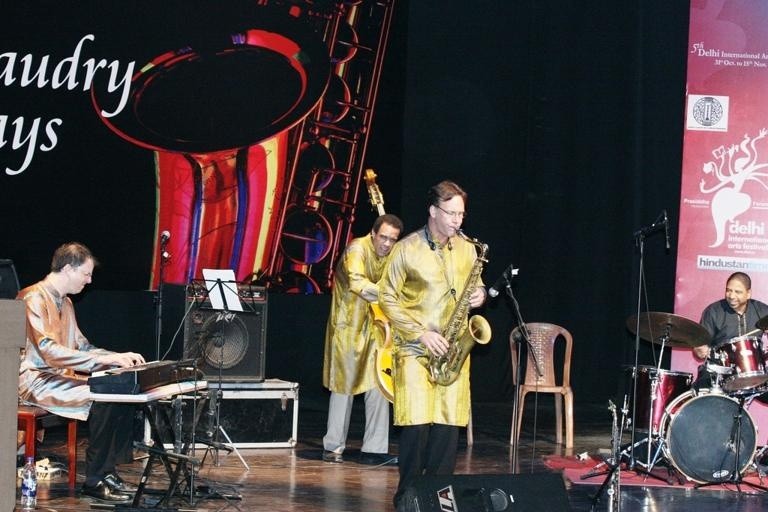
[438,206,467,218]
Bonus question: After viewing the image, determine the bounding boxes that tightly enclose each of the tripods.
[580,238,673,485]
[695,395,768,500]
[198,312,251,470]
[154,365,242,511]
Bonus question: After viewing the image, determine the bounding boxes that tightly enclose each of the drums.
[708,335,765,391]
[625,364,694,435]
[659,388,756,484]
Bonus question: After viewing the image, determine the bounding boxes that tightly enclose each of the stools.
[16,402,80,490]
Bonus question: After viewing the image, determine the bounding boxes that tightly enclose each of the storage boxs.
[142,375,300,449]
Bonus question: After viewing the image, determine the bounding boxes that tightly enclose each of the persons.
[16,243,148,504]
[695,272,768,465]
[375,182,487,510]
[323,214,404,465]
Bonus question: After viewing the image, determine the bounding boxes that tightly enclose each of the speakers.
[394,473,570,512]
[184,285,269,382]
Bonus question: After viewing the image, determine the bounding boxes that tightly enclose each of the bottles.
[21,457,38,498]
[21,495,36,512]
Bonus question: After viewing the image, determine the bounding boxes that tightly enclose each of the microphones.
[192,358,204,365]
[663,209,673,250]
[488,265,516,297]
[159,230,170,245]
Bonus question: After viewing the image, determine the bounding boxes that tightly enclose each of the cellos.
[364,169,395,402]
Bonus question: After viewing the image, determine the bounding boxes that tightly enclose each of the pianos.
[87,358,204,395]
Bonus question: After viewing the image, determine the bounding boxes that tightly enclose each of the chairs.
[509,324,575,449]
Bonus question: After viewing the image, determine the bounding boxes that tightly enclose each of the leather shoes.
[362,453,398,465]
[323,449,343,463]
[103,472,138,495]
[83,480,132,504]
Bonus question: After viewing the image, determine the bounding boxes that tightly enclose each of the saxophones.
[425,225,492,386]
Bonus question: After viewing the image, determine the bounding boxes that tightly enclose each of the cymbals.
[625,312,708,347]
[754,314,768,329]
